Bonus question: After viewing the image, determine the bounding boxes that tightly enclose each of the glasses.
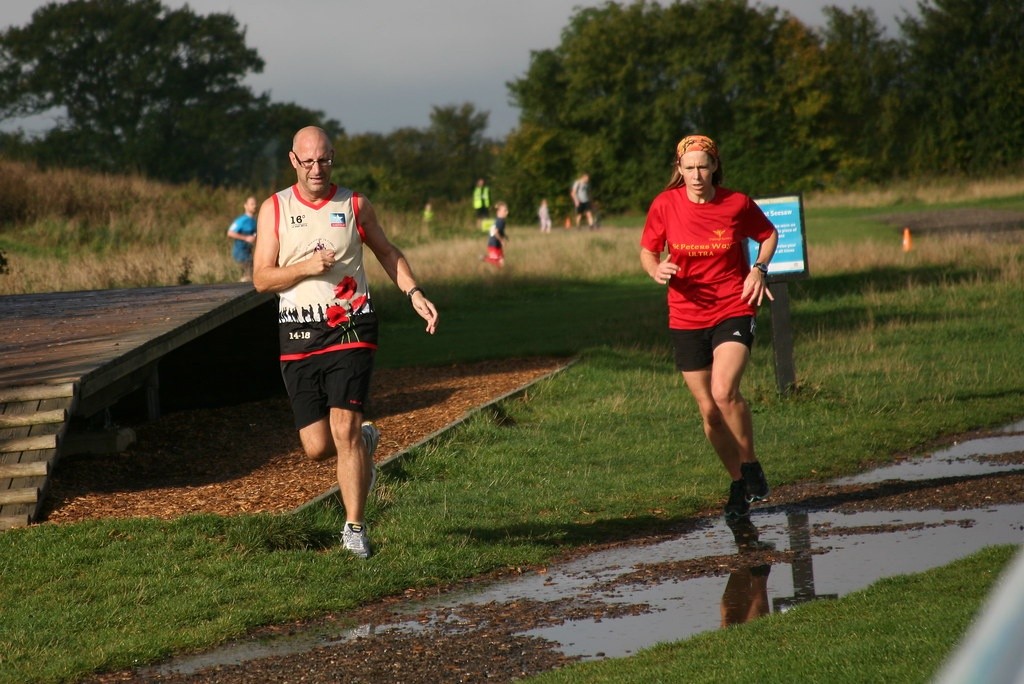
[291,150,335,169]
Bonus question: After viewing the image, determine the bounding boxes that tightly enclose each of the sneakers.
[361,420,381,491]
[722,479,752,519]
[339,522,371,558]
[741,461,771,504]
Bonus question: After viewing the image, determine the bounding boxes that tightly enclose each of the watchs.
[753,263,768,278]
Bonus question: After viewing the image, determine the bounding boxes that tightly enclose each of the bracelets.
[408,287,426,304]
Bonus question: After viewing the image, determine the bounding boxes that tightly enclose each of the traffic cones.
[565,216,572,229]
[901,226,914,253]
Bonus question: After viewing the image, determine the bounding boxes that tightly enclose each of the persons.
[252,125,442,558]
[228,196,258,285]
[471,177,492,229]
[482,199,510,269]
[423,203,436,224]
[570,174,597,231]
[538,198,553,235]
[637,135,780,521]
